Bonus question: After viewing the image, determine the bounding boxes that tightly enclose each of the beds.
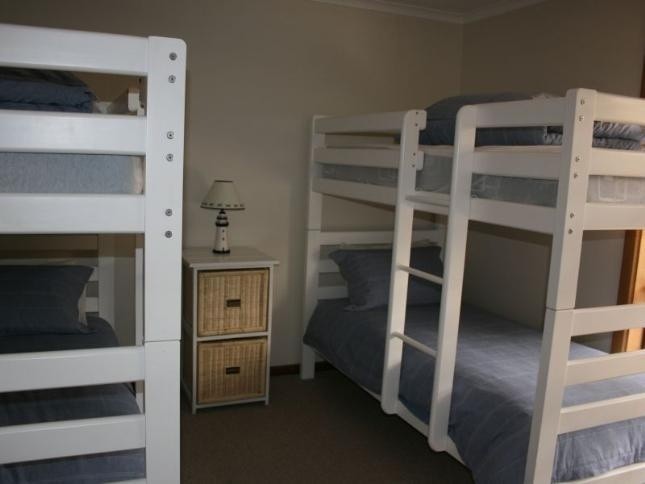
[301,88,645,484]
[0,23,186,483]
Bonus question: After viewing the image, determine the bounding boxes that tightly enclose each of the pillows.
[328,240,446,314]
[0,265,96,335]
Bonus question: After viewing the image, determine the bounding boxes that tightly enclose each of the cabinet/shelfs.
[179,246,280,415]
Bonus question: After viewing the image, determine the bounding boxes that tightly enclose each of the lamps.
[200,180,246,255]
